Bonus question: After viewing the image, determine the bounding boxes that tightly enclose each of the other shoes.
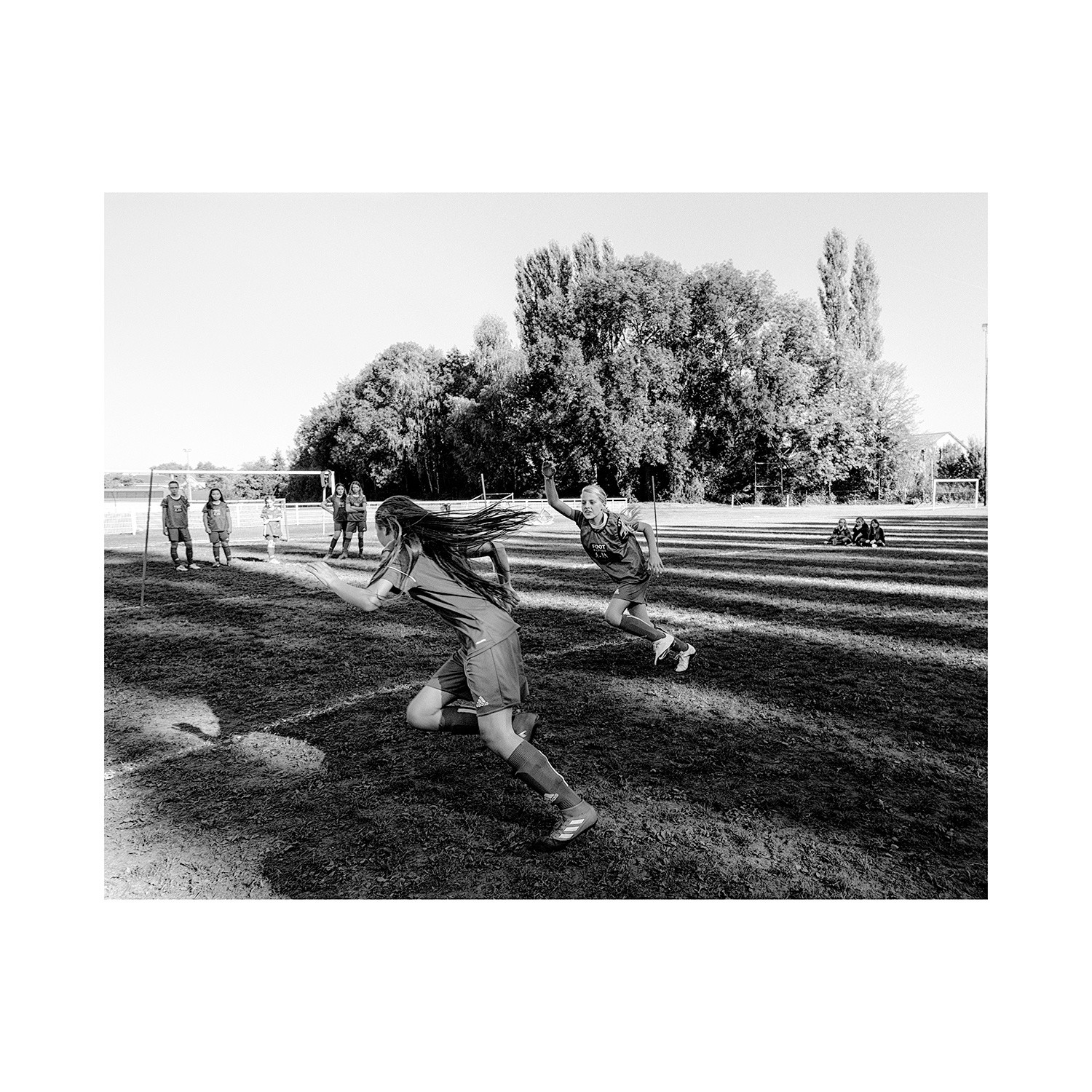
[213,562,220,567]
[188,563,200,570]
[339,553,348,560]
[227,560,233,567]
[359,554,365,559]
[269,559,280,564]
[176,564,188,572]
[323,553,332,559]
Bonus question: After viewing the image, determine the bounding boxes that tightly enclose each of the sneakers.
[541,800,598,847]
[651,632,676,666]
[675,643,697,673]
[511,713,539,742]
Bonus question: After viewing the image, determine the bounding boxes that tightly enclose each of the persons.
[861,519,886,547]
[160,481,201,572]
[824,518,851,545]
[847,517,870,547]
[305,495,599,842]
[320,483,348,559]
[260,495,282,564]
[542,460,696,673]
[201,488,232,566]
[337,482,368,559]
